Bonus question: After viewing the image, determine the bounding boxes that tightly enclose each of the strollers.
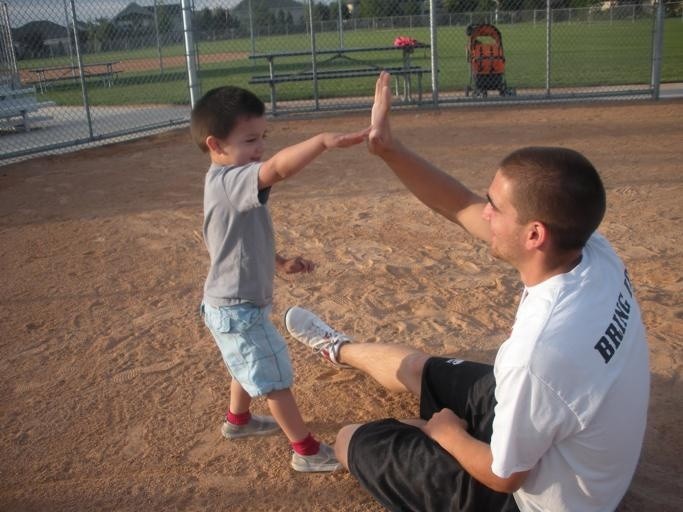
[465,23,514,97]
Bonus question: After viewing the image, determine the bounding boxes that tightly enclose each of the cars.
[574,1,640,21]
[640,1,682,18]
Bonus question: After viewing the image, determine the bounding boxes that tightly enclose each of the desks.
[248,42,430,116]
[28,58,120,95]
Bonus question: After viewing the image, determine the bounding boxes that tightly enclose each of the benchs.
[0,70,124,134]
[247,65,440,103]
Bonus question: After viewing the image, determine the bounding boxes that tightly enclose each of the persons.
[284,62,650,512]
[187,87,373,471]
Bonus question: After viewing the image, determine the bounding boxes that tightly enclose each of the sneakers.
[290,442,346,472]
[283,306,355,370]
[221,413,281,439]
